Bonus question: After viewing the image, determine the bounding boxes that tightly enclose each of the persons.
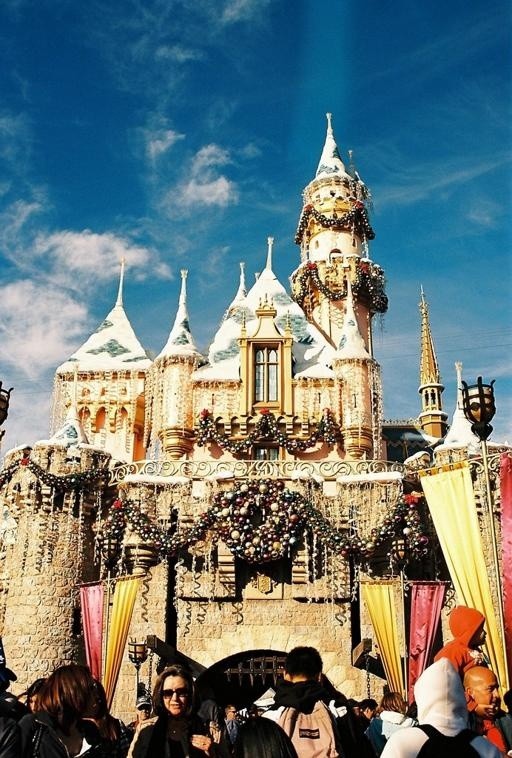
[2,646,512,757]
[433,605,501,720]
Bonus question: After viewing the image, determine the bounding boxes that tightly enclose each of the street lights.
[124,638,149,696]
[455,373,512,686]
[0,379,15,442]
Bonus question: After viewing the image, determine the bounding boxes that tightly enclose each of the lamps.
[127,639,149,693]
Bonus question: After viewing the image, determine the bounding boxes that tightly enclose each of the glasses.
[160,688,187,697]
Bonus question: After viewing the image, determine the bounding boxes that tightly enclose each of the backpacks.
[279,700,342,758]
